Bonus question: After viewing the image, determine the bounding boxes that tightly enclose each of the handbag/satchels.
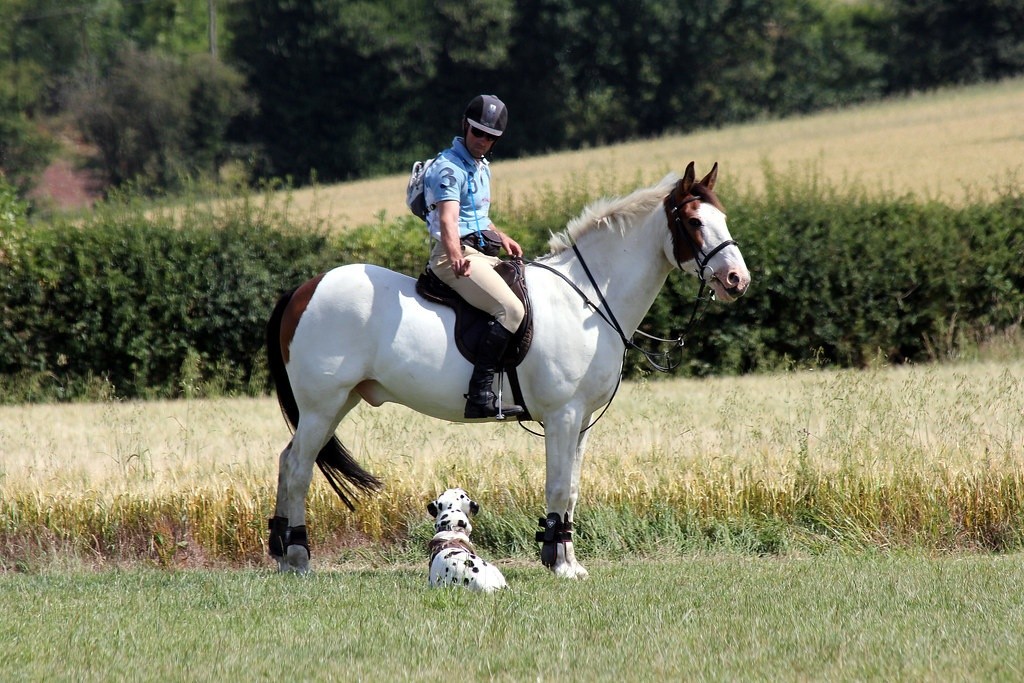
[466,230,501,257]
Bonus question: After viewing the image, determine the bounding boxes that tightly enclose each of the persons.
[423,95,527,422]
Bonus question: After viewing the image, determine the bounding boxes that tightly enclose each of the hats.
[463,94,509,136]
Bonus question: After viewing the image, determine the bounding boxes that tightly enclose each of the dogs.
[427,488,511,593]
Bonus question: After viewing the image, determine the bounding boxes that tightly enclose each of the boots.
[463,333,523,418]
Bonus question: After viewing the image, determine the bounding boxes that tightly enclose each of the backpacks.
[405,156,435,221]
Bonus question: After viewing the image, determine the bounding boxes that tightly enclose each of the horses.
[264,160,753,579]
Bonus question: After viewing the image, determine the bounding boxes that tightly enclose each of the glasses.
[469,124,498,141]
